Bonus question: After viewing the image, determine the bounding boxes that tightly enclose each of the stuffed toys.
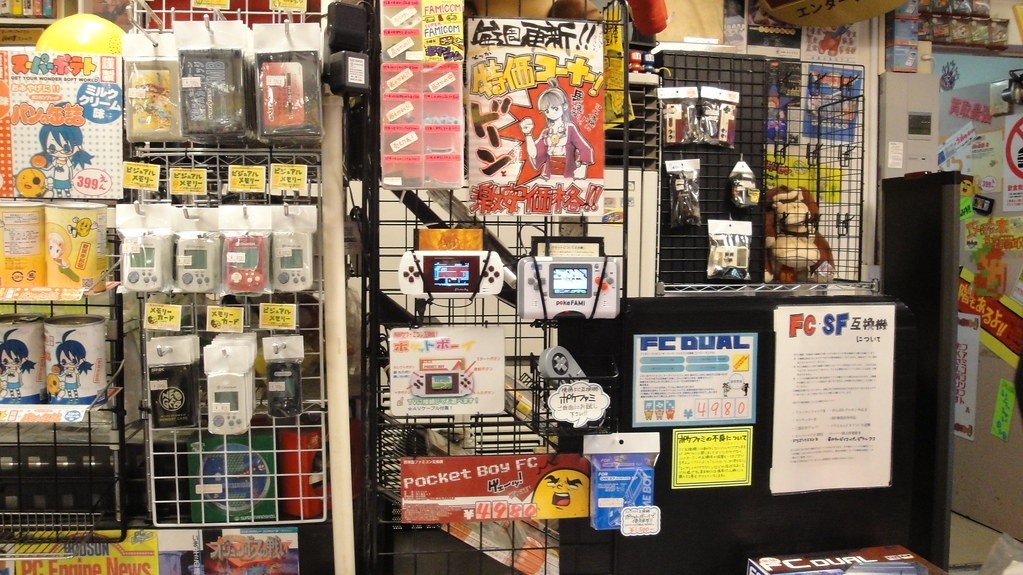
[765,185,835,283]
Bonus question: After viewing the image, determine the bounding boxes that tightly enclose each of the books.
[441,519,559,575]
